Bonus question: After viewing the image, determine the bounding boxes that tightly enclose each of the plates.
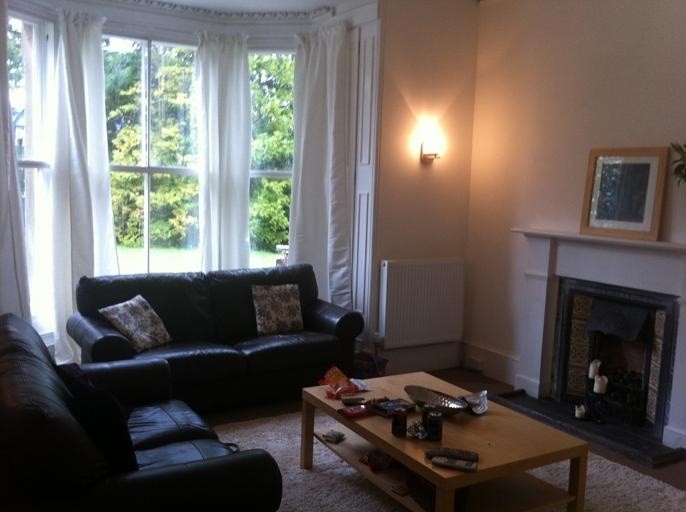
[403,384,470,420]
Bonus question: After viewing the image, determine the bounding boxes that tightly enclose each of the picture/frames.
[579,145,671,243]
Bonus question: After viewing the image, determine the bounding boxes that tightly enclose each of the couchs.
[65,264,364,417]
[0,314,284,512]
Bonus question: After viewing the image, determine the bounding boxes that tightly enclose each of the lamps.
[420,127,446,163]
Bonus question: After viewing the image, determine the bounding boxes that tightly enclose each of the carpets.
[211,405,686,511]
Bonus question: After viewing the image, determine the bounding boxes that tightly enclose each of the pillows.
[98,294,171,354]
[252,284,304,334]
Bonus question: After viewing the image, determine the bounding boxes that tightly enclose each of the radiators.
[380,256,463,349]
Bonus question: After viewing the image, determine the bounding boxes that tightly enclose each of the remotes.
[426,445,479,462]
[431,455,478,472]
